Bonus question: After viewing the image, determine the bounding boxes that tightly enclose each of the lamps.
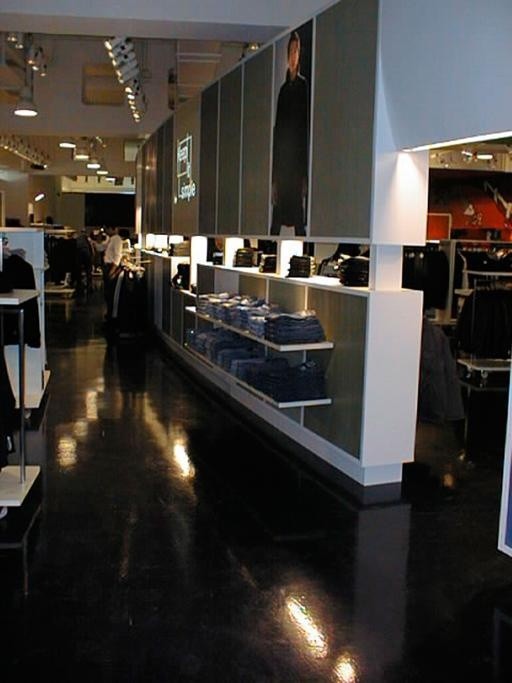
[0,33,149,174]
[476,150,497,161]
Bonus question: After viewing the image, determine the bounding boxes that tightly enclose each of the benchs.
[45,286,75,323]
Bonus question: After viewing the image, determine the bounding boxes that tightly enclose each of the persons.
[118,229,131,263]
[76,227,95,287]
[90,226,105,273]
[90,220,124,319]
[0,237,42,349]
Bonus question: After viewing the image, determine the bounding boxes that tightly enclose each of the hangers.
[2,245,12,258]
[475,278,498,290]
[463,247,488,251]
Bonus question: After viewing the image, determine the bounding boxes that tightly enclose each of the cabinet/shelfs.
[176,287,334,409]
